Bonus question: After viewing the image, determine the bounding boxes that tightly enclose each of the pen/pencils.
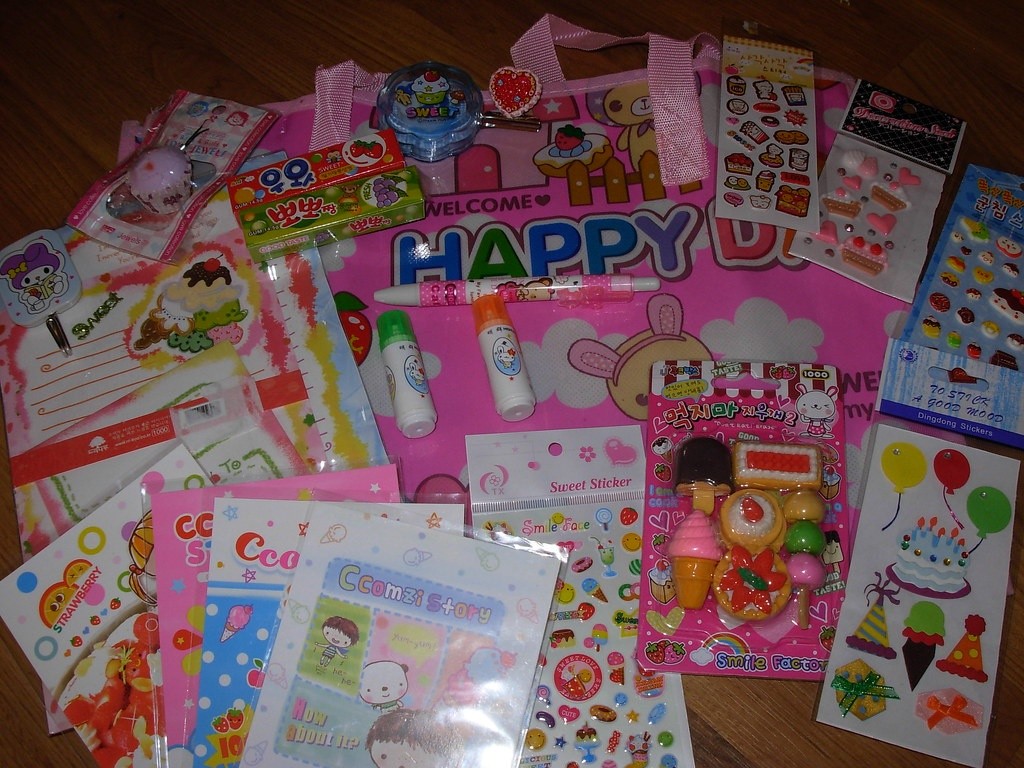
[372,271,662,310]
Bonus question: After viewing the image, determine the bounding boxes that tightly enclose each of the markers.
[375,309,440,440]
[469,294,537,424]
